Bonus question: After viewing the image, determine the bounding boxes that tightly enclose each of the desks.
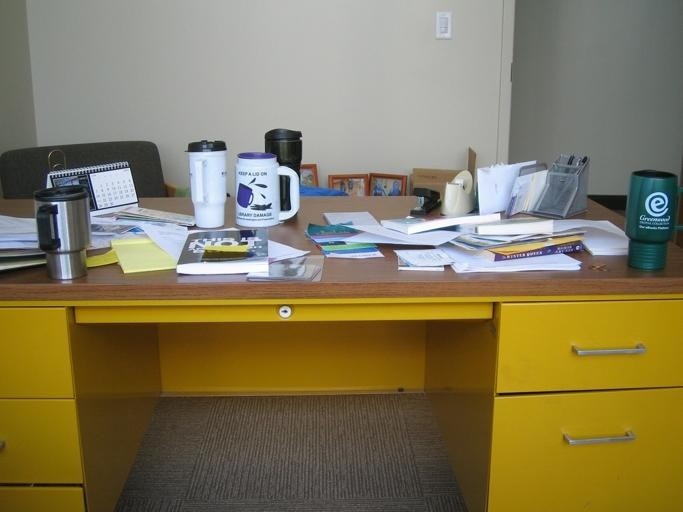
[0,191,681,512]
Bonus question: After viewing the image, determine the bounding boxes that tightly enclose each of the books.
[0,205,629,281]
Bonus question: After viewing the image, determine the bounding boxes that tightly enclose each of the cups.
[184,141,231,229]
[33,186,89,281]
[628,170,678,270]
[235,152,298,226]
[265,129,303,218]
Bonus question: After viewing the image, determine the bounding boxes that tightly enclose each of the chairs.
[2,137,169,198]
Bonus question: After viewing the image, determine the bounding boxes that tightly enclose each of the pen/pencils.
[554,155,592,206]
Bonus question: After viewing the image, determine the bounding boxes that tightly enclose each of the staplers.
[409,188,441,216]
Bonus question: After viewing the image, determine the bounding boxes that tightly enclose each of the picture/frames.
[298,163,409,198]
